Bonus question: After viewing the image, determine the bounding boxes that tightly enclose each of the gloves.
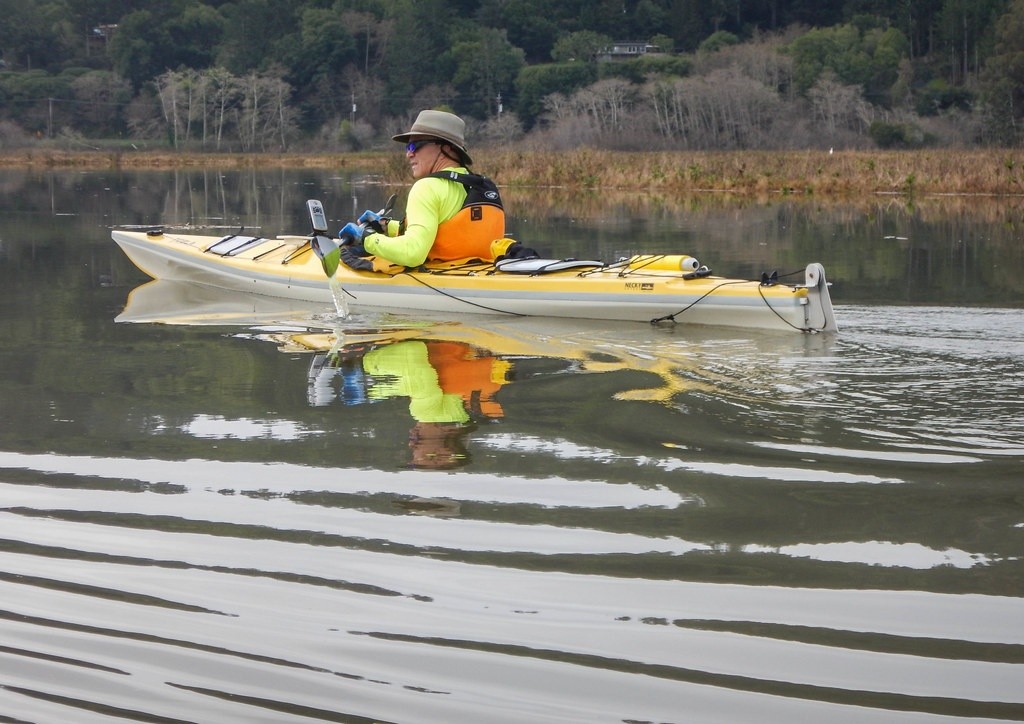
[338,370,366,405]
[357,209,381,226]
[338,222,365,242]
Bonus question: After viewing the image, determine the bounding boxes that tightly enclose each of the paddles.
[311,192,399,278]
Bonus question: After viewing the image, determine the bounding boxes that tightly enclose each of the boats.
[108,229,838,337]
[112,278,828,371]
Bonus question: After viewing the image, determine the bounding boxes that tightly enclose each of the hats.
[391,110,474,166]
[396,452,473,469]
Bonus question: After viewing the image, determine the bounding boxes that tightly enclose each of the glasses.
[406,139,453,153]
[407,436,457,443]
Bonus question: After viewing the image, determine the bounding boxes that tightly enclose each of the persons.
[339,110,505,267]
[326,341,516,472]
